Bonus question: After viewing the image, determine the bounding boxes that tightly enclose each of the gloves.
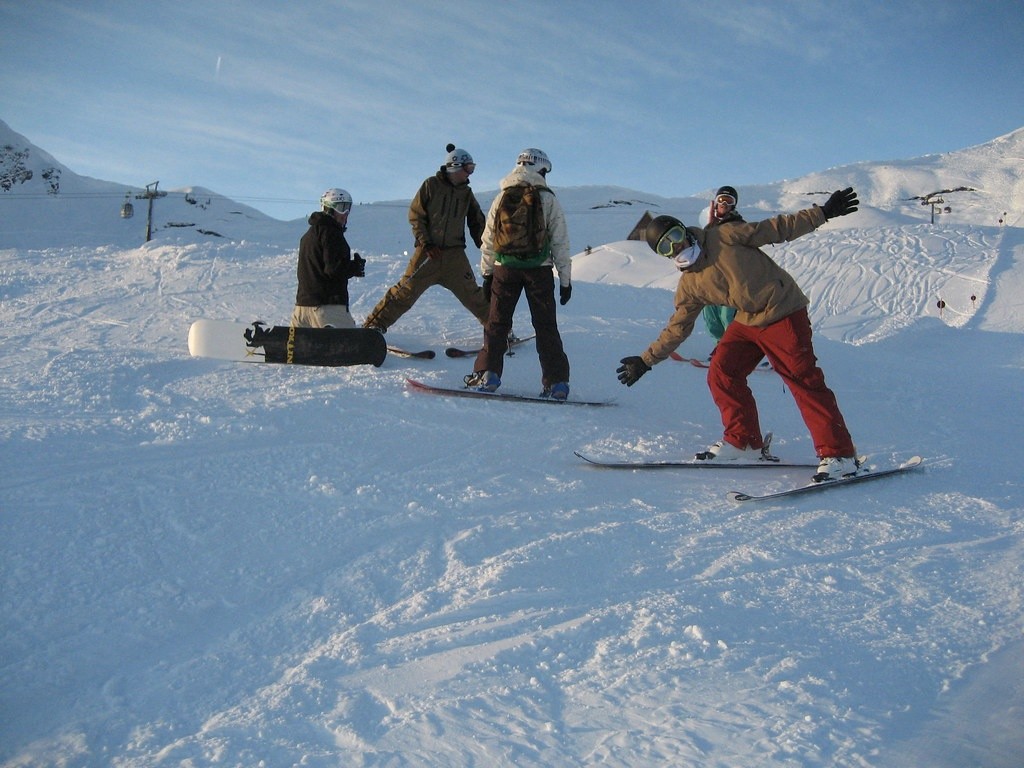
[350,253,367,278]
[819,186,860,222]
[482,275,493,303]
[560,283,573,305]
[615,355,652,387]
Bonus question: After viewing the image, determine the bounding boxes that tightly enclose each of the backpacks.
[493,184,556,259]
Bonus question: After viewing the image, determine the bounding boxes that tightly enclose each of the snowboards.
[188,318,388,368]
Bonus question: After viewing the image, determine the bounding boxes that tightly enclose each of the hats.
[444,148,473,173]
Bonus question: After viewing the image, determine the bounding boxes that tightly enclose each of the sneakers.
[539,382,570,400]
[507,330,519,340]
[818,456,864,482]
[710,438,764,461]
[462,371,502,393]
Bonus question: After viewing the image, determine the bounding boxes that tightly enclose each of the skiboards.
[573,447,923,506]
[672,349,774,373]
[389,328,540,360]
[406,374,619,406]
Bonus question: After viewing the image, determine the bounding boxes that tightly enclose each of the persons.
[362,148,518,347]
[615,186,861,484]
[697,185,747,362]
[290,187,367,327]
[462,147,573,399]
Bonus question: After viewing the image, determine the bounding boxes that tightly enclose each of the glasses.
[447,162,476,174]
[332,201,352,212]
[655,225,685,257]
[715,194,736,205]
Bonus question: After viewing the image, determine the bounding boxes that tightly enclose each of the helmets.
[716,186,739,204]
[646,214,684,254]
[515,149,552,172]
[320,188,353,215]
